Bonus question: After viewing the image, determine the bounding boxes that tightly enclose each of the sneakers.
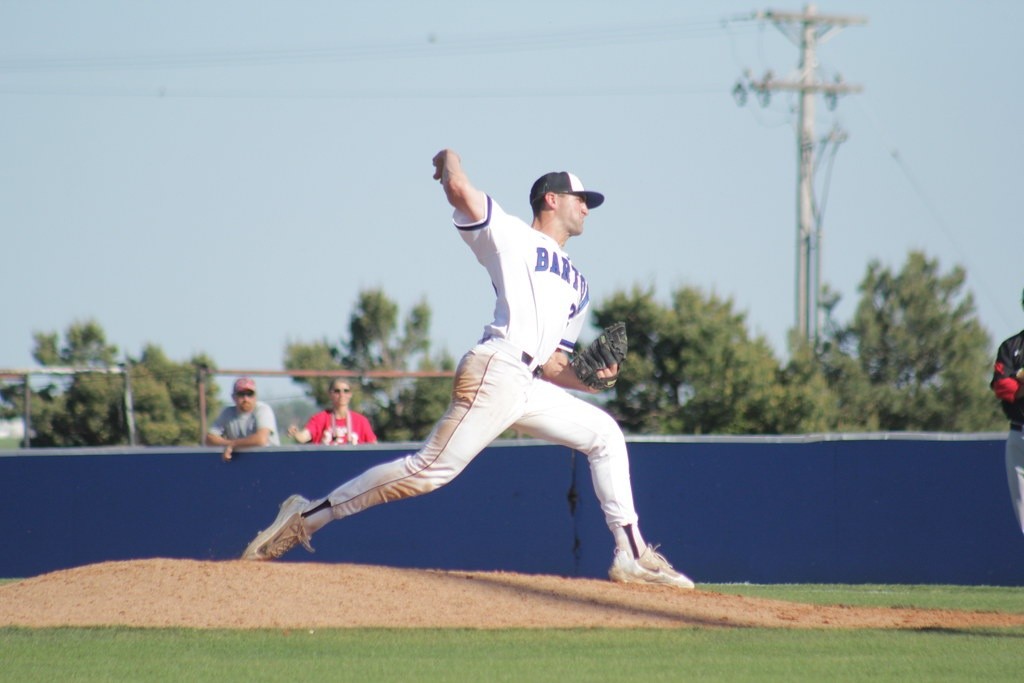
[608,542,695,591]
[239,493,316,561]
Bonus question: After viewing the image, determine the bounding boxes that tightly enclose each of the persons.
[241,149,696,589]
[287,376,379,445]
[988,289,1024,534]
[205,377,280,461]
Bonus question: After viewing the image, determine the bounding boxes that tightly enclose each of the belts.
[485,336,544,378]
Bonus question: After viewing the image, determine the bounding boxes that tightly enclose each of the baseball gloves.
[569,321,628,391]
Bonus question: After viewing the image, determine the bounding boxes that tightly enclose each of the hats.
[529,171,605,209]
[231,379,256,396]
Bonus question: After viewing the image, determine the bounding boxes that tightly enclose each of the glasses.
[237,390,254,398]
[332,389,351,393]
